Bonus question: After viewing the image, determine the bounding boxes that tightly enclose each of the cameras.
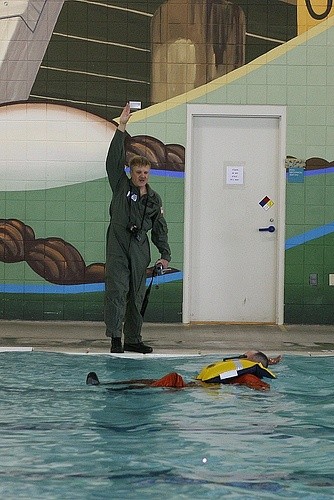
[126,221,141,234]
[155,259,171,276]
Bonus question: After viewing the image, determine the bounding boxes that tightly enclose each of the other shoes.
[127,343,152,353]
[111,344,124,353]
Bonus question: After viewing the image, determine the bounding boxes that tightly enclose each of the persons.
[84,348,282,405]
[103,100,171,354]
